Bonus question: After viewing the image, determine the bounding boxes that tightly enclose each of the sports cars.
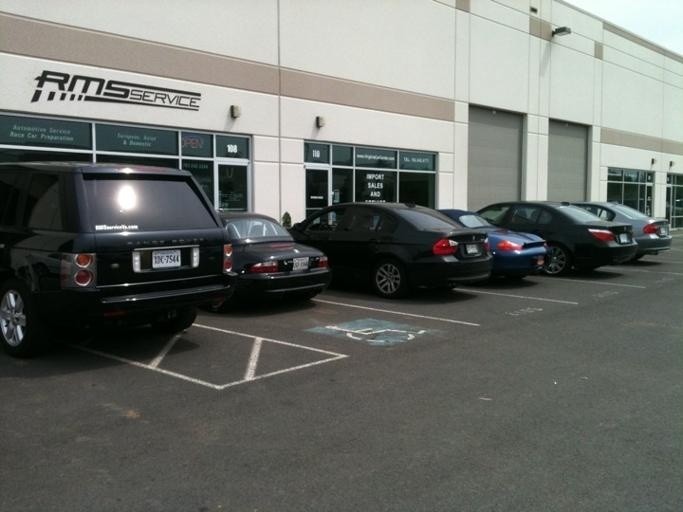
[437,208,549,288]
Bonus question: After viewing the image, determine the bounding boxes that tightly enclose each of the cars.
[208,211,331,316]
[472,201,638,278]
[562,201,672,264]
[288,201,499,300]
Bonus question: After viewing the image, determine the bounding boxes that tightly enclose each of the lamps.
[551,27,571,37]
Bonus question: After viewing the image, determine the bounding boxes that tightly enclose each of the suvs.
[0,161,237,359]
